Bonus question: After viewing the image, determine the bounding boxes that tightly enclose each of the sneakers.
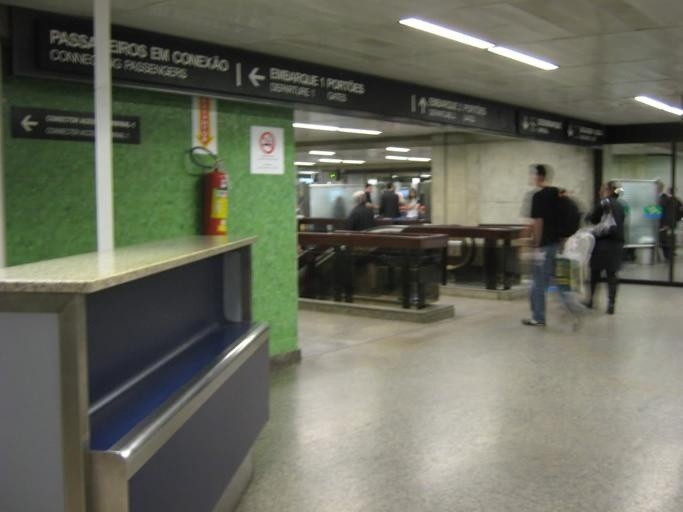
[521,318,546,327]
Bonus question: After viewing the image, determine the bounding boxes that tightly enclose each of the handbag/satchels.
[586,217,617,236]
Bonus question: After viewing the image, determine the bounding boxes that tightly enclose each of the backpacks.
[553,195,580,238]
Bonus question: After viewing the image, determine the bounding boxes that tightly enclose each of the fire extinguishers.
[189,145,228,236]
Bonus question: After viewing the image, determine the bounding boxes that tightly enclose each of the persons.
[580,180,628,314]
[656,177,676,262]
[332,180,428,231]
[521,164,561,327]
[666,185,682,223]
[557,188,569,254]
